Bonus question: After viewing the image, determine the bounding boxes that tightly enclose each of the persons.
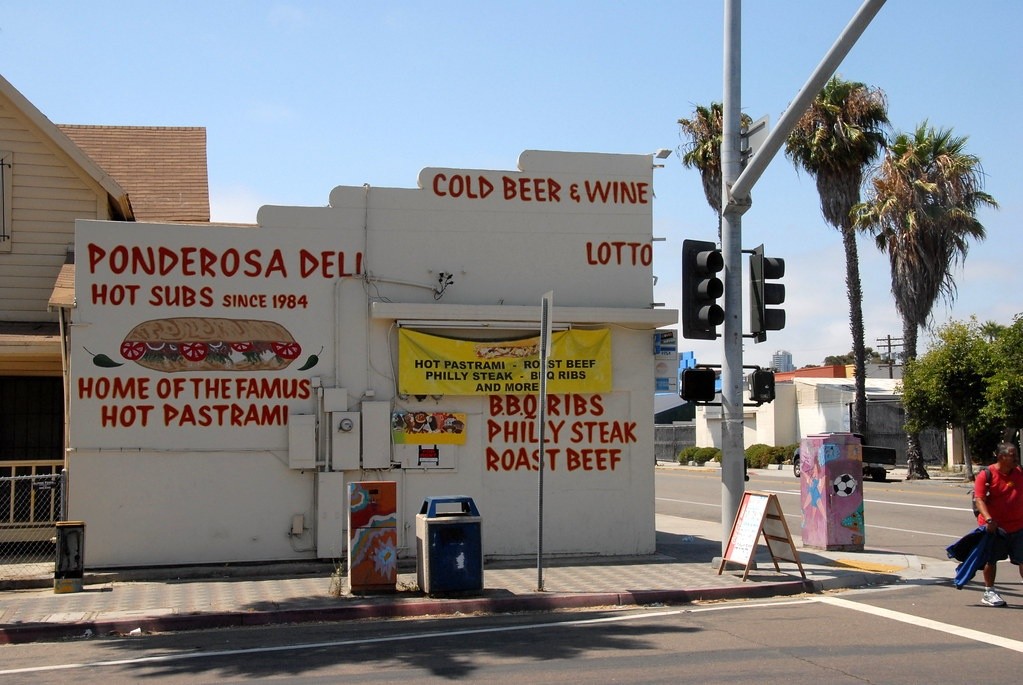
[972,442,1023,606]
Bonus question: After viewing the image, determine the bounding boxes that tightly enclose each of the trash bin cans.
[414,496,485,599]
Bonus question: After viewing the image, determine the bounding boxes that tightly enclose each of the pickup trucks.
[791,430,896,482]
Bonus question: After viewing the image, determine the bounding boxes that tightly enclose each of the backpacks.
[972,466,991,516]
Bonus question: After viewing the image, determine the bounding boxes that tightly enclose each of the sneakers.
[981,590,1006,606]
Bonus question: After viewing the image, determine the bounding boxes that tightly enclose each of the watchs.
[984,518,994,524]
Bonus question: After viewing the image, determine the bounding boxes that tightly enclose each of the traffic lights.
[682,368,715,401]
[681,238,725,340]
[749,244,786,343]
[751,369,776,403]
